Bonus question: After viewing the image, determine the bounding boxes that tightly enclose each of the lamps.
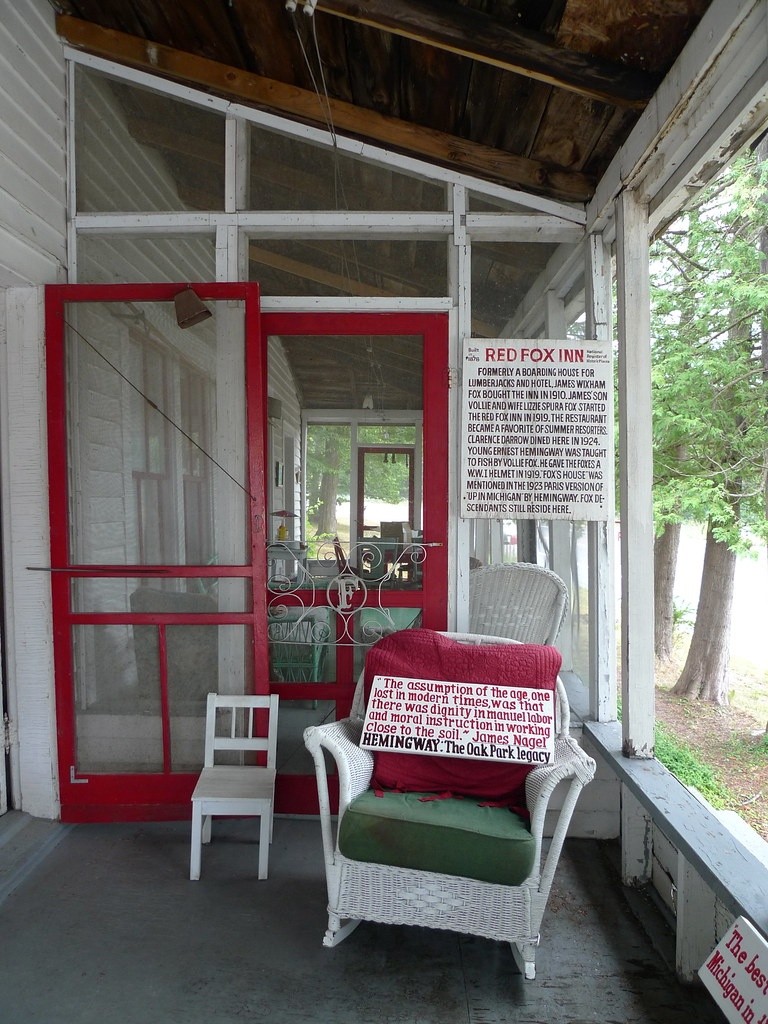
[362,363,374,409]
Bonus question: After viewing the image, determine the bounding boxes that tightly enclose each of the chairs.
[401,522,423,582]
[470,562,569,646]
[129,587,218,699]
[189,693,280,881]
[357,537,398,590]
[269,611,332,710]
[303,629,596,980]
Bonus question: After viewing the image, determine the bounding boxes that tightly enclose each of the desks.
[267,547,309,584]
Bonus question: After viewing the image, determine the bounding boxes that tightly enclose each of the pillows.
[363,628,564,817]
